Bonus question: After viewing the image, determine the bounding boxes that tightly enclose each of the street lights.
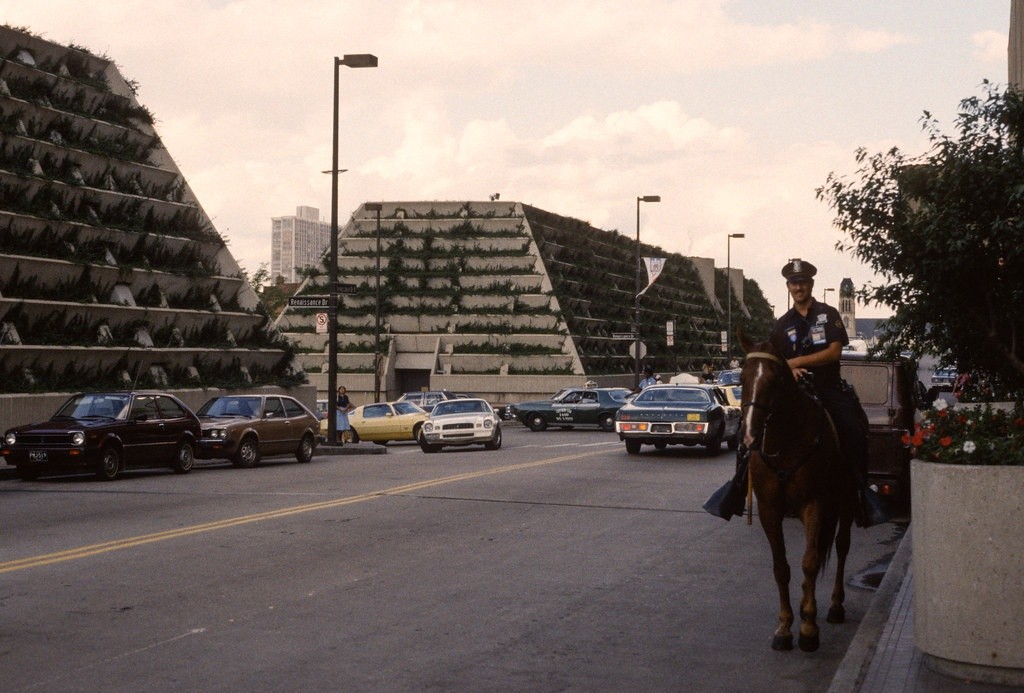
[633,195,661,389]
[823,287,834,303]
[328,54,379,445]
[727,232,745,370]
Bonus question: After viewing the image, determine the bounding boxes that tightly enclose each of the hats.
[641,365,653,371]
[782,262,817,278]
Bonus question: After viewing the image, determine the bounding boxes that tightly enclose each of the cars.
[510,386,638,432]
[0,391,203,480]
[320,400,429,444]
[614,370,747,451]
[395,390,502,453]
[931,366,958,390]
[196,394,322,468]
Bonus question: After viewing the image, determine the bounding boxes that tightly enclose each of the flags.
[636,257,666,299]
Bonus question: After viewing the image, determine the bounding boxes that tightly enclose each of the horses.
[732,318,860,650]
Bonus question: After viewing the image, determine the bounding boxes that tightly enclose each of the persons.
[729,356,740,368]
[337,386,351,446]
[701,363,715,385]
[623,367,656,401]
[655,374,663,385]
[721,261,888,528]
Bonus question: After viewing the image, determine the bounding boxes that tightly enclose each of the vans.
[838,348,930,511]
